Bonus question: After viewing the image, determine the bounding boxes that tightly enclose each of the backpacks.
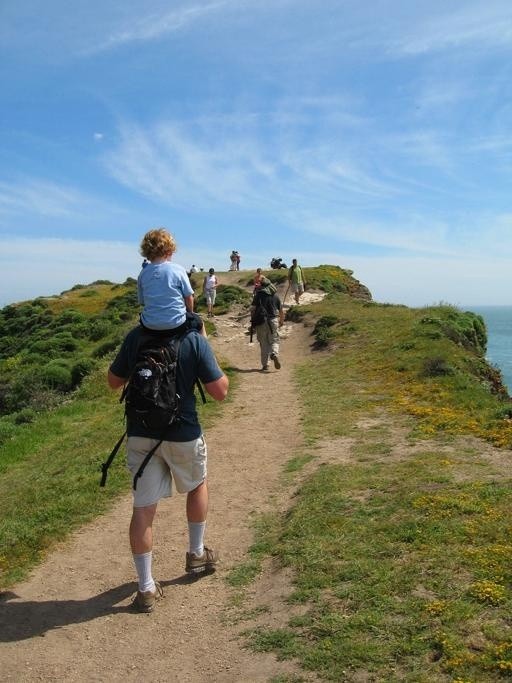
[124,329,199,433]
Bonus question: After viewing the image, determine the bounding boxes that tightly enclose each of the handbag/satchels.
[253,304,268,326]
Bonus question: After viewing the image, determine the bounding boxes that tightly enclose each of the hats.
[256,278,277,296]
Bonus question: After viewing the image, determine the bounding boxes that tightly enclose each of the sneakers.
[207,311,214,318]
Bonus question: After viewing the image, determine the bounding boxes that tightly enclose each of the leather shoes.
[263,352,281,370]
[185,545,224,574]
[135,580,169,613]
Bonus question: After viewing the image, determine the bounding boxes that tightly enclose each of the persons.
[288,259,305,305]
[252,277,284,373]
[138,228,208,337]
[190,265,196,272]
[203,268,217,318]
[230,251,240,271]
[200,267,204,272]
[107,296,229,613]
[271,258,287,269]
[254,268,265,289]
[142,259,148,268]
